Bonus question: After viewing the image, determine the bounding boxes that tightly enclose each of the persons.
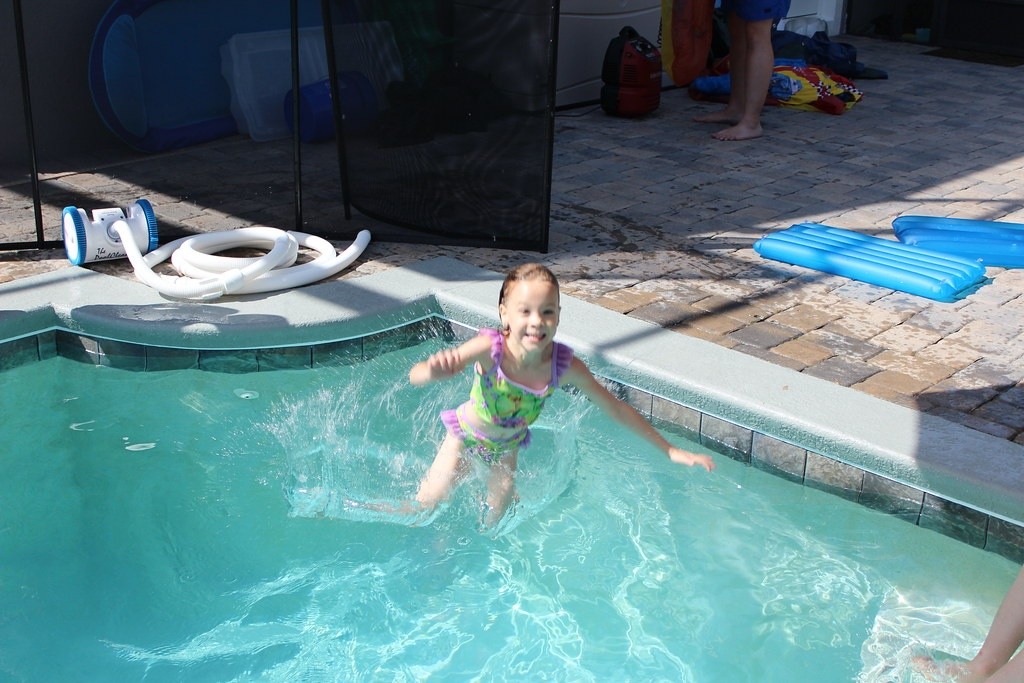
[693,0,790,142]
[408,262,716,508]
[911,563,1024,683]
